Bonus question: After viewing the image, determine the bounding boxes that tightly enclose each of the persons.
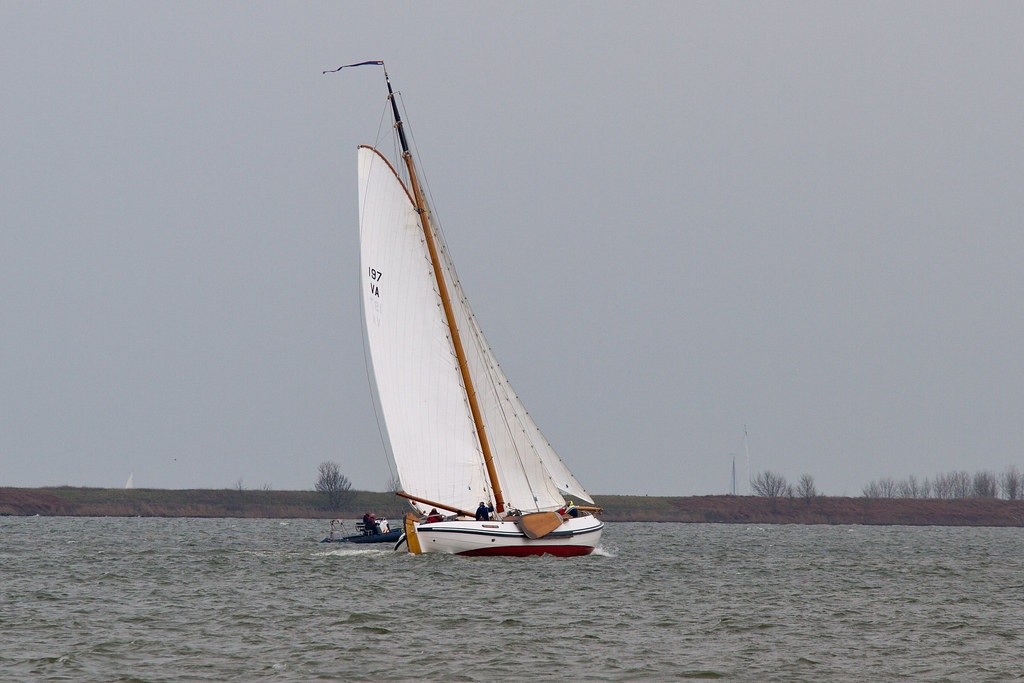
[476,502,494,520]
[362,511,375,533]
[426,509,444,522]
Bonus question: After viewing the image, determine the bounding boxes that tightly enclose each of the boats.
[320,526,406,543]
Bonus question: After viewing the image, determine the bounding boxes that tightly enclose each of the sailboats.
[322,59,604,557]
